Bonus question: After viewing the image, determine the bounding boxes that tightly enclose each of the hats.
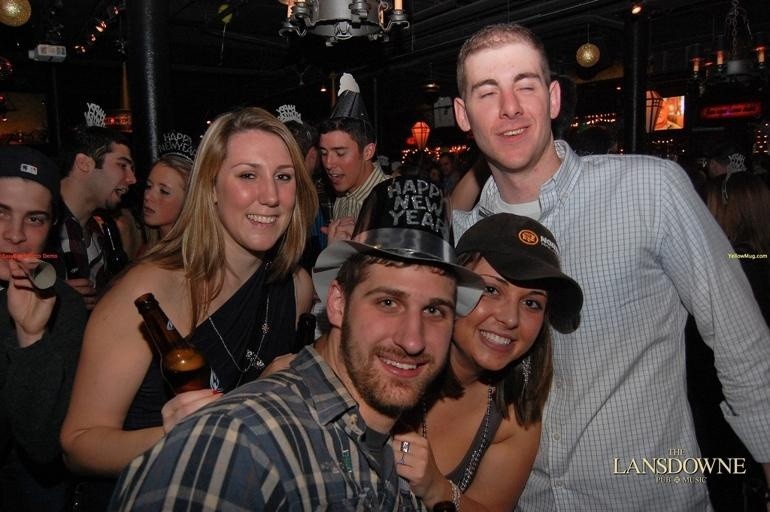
[339,174,490,289]
[0,154,56,196]
[327,72,369,123]
[455,213,583,333]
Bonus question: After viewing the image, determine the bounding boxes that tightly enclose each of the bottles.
[100,221,129,273]
[292,312,317,355]
[132,291,227,397]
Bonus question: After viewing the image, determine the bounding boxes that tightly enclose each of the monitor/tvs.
[653,94,687,131]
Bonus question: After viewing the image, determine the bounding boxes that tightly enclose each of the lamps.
[411,121,431,150]
[687,0,769,102]
[276,0,413,47]
[0,0,34,28]
[575,19,601,68]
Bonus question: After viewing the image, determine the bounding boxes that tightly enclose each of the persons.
[0,22,770,512]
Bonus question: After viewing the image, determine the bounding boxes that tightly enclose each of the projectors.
[34,44,66,63]
